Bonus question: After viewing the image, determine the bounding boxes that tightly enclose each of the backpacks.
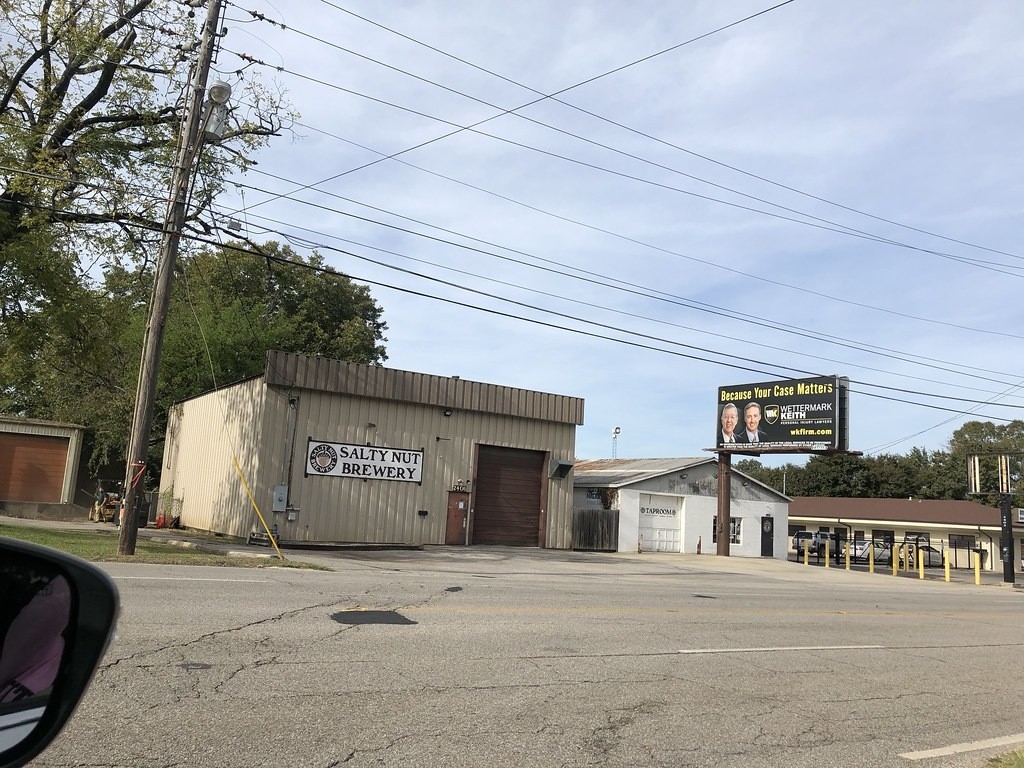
[105,494,110,504]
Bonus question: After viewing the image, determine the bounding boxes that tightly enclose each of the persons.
[738,402,771,442]
[92,486,109,523]
[717,402,746,448]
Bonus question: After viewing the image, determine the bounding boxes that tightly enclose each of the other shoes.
[729,439,733,443]
[94,521,98,523]
[104,520,106,523]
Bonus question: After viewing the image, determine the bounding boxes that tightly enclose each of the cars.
[841,540,891,563]
[899,544,945,567]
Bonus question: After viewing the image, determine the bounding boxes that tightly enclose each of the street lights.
[115,80,232,559]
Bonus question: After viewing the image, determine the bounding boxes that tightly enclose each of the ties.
[753,437,757,443]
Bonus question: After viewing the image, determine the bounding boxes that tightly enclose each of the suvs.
[792,531,848,558]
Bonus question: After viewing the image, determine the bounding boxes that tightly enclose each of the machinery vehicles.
[81,478,122,522]
